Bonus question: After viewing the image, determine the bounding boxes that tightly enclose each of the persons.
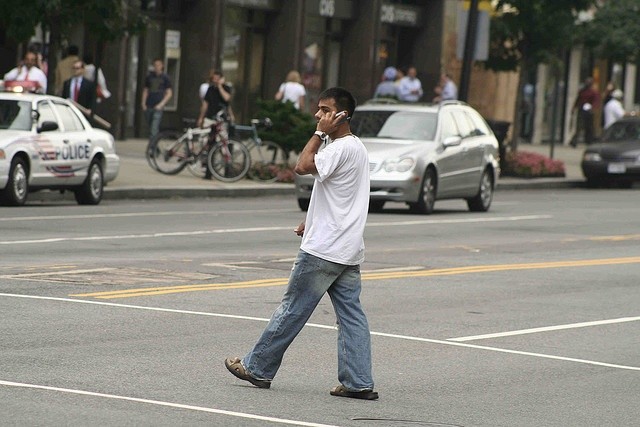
[275,70,306,112]
[82,55,111,98]
[604,89,640,130]
[432,86,443,104]
[602,82,617,127]
[3,49,47,94]
[224,88,379,400]
[569,77,602,147]
[141,59,172,157]
[395,67,423,102]
[56,45,85,88]
[63,62,96,123]
[197,71,232,180]
[373,66,398,98]
[200,69,235,101]
[441,73,458,100]
[19,42,48,75]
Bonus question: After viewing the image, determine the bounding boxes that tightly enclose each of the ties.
[73,79,78,102]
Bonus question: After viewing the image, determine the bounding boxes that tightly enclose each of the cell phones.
[333,112,350,125]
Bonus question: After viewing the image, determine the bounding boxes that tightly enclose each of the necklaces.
[332,132,353,141]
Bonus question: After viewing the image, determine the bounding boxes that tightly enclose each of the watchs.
[314,131,329,141]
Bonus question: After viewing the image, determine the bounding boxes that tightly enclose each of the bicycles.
[187,118,289,183]
[146,109,251,182]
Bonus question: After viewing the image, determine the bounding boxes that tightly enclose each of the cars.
[293,97,501,214]
[582,111,640,188]
[0,81,120,206]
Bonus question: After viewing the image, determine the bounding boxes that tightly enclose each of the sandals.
[224,357,272,391]
[330,384,380,402]
